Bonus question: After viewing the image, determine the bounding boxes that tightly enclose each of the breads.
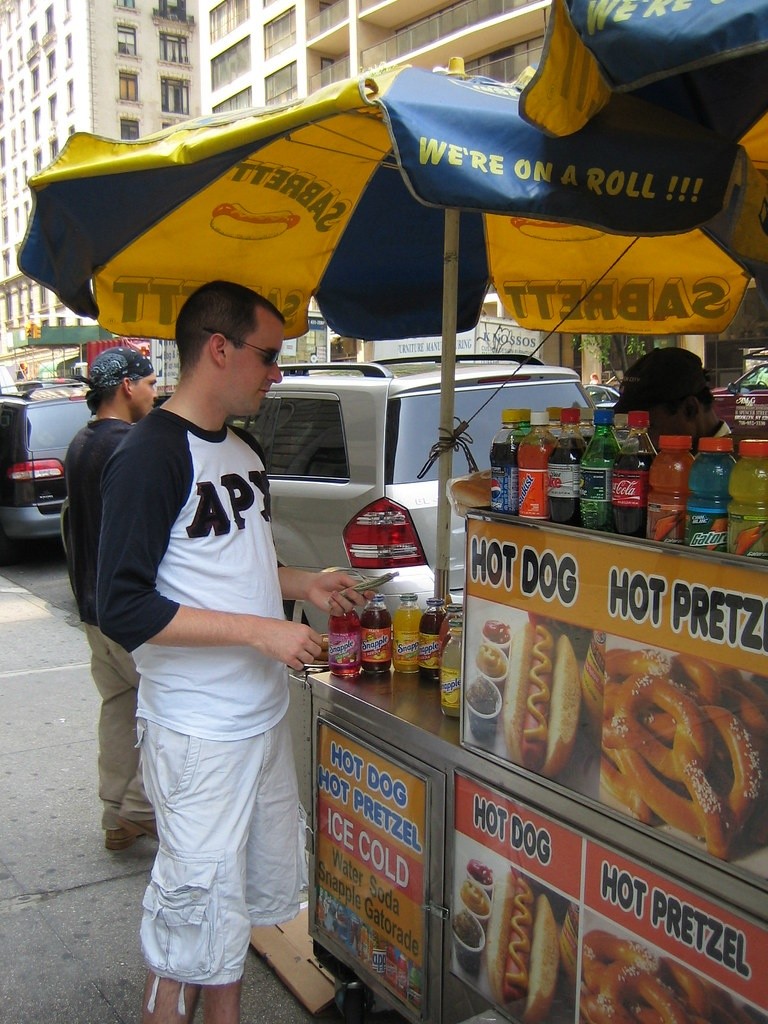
[600,646,768,862]
[578,929,768,1024]
[451,469,491,508]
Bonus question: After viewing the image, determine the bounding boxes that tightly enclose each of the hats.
[610,347,707,413]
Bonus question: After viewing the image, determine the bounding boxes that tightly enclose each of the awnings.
[15,322,111,363]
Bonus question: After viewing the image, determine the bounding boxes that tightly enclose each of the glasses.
[202,327,279,366]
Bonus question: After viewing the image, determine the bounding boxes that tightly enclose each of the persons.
[609,346,740,444]
[96,280,380,1024]
[60,346,156,849]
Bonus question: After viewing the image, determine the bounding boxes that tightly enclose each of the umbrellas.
[17,53,768,623]
[516,0,768,173]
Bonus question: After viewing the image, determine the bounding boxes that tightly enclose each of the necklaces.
[95,416,123,420]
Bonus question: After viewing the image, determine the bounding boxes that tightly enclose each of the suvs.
[154,354,598,672]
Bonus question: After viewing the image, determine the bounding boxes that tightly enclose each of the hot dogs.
[502,621,581,772]
[485,868,560,1024]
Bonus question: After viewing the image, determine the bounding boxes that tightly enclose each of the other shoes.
[116,812,158,842]
[104,825,144,850]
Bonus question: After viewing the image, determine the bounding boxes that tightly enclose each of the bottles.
[645,435,695,544]
[439,603,463,656]
[360,594,392,673]
[727,439,768,560]
[440,619,462,655]
[419,597,447,678]
[393,593,422,674]
[489,407,660,537]
[684,437,737,553]
[329,599,361,676]
[440,626,463,717]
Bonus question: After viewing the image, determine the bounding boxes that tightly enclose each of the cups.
[465,677,503,744]
[451,860,495,976]
[481,627,513,656]
[473,648,508,692]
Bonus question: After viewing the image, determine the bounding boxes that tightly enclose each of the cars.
[709,362,768,441]
[0,374,93,568]
[582,384,622,409]
